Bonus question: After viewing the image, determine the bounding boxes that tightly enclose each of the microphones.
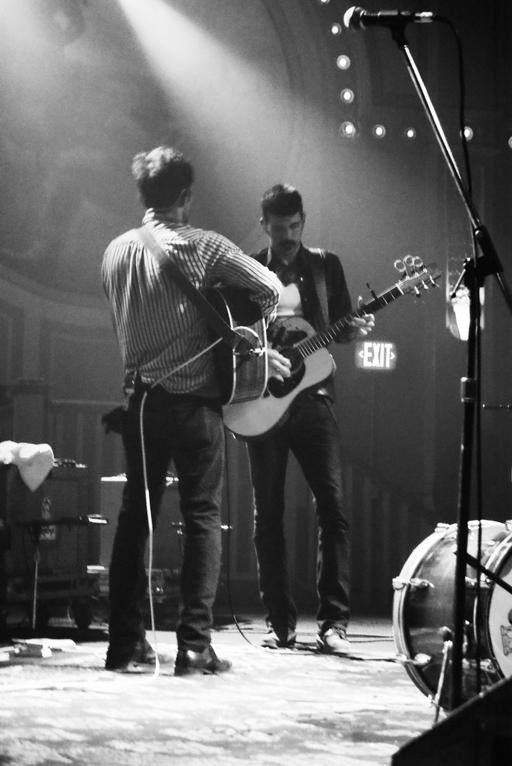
[343,6,434,33]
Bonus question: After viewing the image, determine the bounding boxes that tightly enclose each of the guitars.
[209,288,268,405]
[222,255,442,442]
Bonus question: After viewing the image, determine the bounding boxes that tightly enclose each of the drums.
[393,519,512,712]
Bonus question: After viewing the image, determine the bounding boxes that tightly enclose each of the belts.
[307,385,337,404]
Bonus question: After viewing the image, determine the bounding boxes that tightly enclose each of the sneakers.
[316,628,353,654]
[259,624,298,651]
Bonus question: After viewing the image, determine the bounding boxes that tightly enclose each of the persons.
[218,182,377,660]
[99,144,284,679]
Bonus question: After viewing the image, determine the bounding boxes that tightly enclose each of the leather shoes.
[174,645,231,676]
[105,640,172,669]
